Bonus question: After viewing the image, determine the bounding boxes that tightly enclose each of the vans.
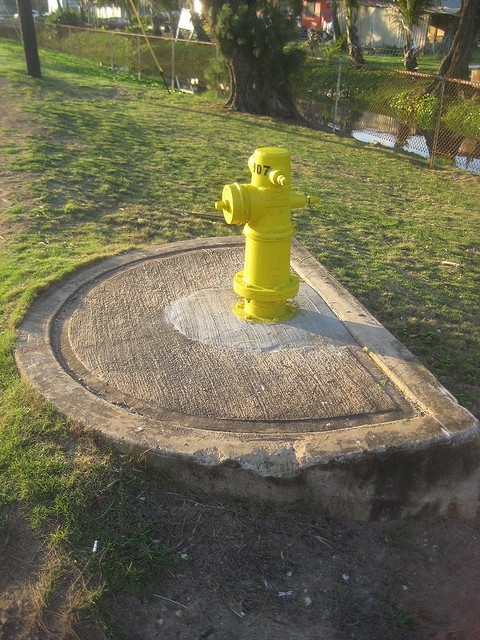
[106,18,130,31]
[256,0,336,43]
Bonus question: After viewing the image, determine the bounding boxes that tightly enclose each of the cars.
[14,10,39,19]
[42,13,49,17]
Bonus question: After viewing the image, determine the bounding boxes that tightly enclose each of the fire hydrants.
[215,147,313,325]
